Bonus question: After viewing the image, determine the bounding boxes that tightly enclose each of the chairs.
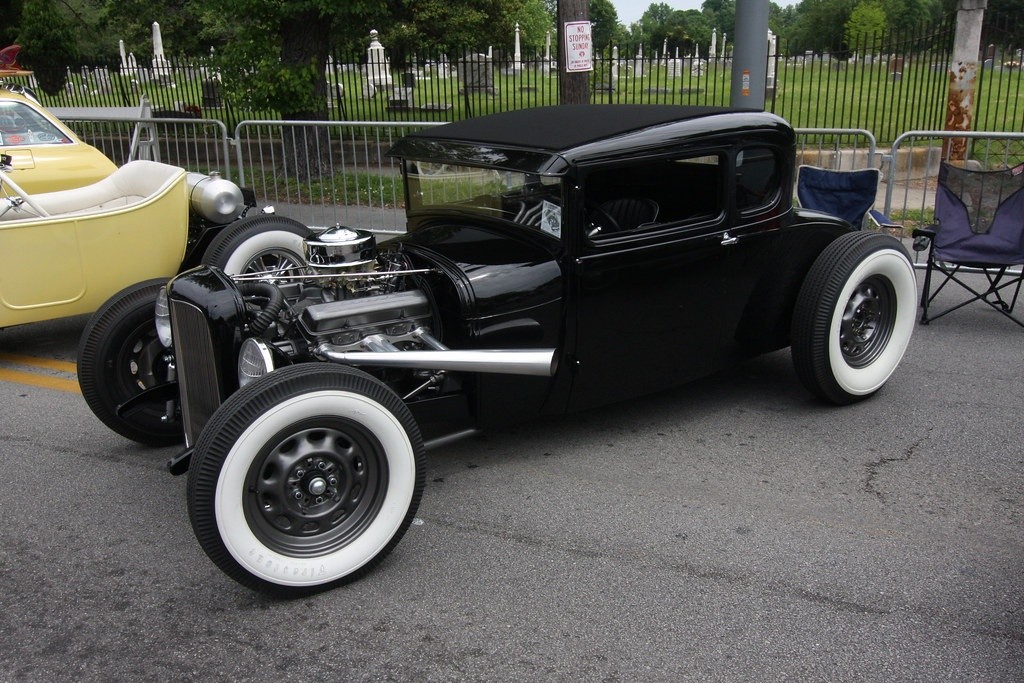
[591,198,659,233]
[796,164,904,232]
[68,160,185,214]
[912,157,1024,329]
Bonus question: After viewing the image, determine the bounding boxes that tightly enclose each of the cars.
[0,44,276,344]
[75,104,918,600]
